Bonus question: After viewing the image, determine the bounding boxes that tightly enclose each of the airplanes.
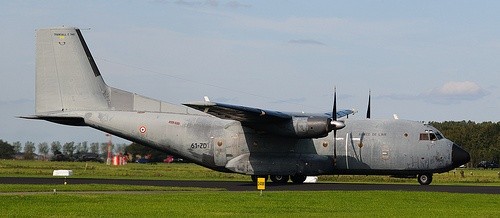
[15,24,471,187]
